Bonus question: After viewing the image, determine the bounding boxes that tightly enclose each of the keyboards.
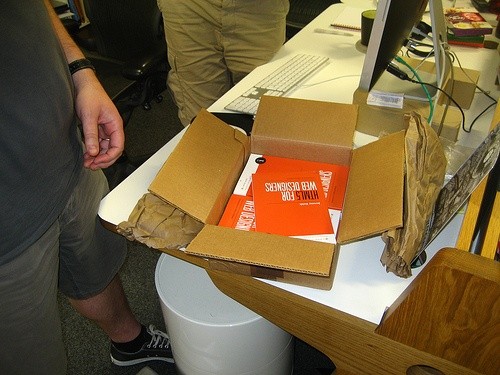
[224,54,330,115]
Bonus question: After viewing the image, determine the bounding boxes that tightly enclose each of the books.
[444,6,493,37]
[218,153,350,245]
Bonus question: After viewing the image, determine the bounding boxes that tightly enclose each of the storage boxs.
[148,95,405,290]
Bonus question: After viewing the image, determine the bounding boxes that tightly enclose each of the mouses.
[230,125,247,136]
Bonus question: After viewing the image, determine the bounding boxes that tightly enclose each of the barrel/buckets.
[155,253,293,375]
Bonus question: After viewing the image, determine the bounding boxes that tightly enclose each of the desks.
[98,3,500,375]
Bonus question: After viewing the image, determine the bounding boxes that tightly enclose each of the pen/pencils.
[315,30,354,36]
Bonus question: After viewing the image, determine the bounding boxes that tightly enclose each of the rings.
[105,139,110,141]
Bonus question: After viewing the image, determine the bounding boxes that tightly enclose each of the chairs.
[74,0,170,116]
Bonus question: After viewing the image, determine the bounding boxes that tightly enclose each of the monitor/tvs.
[352,0,480,147]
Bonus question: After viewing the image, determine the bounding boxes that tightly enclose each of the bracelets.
[68,58,96,76]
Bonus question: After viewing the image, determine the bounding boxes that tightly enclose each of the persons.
[0,0,176,375]
[157,0,290,128]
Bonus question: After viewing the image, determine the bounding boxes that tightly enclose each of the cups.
[361,10,377,47]
